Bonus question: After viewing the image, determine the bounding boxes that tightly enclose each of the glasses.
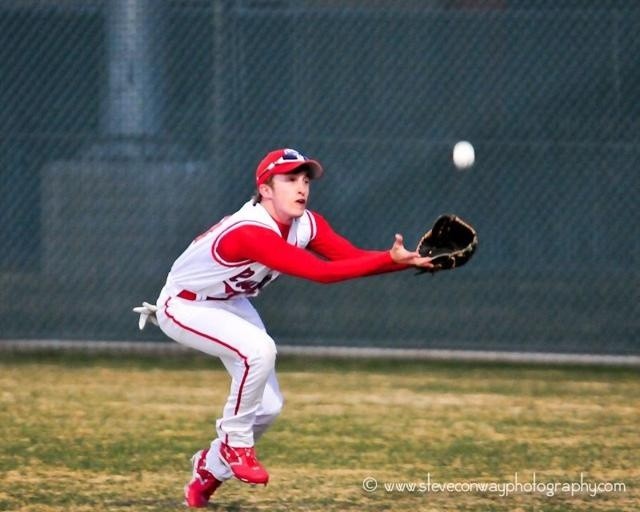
[258,154,309,179]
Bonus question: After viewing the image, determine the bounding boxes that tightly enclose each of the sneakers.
[184,448,223,507]
[218,443,268,485]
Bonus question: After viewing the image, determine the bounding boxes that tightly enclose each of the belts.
[177,290,196,300]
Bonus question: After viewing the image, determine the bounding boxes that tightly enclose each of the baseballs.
[452,141,476,170]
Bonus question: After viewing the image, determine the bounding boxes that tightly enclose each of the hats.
[256,148,323,190]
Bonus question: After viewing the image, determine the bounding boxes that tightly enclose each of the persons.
[154,144,479,509]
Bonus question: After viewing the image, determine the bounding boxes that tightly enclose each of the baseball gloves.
[413,214,479,276]
